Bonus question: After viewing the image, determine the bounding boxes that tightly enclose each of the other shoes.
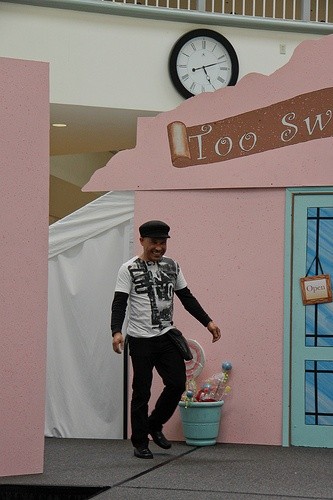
[134,447,154,459]
[148,430,171,449]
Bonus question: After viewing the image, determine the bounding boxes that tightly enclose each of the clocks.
[170,29,239,99]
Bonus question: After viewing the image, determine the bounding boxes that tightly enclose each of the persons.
[111,220,221,459]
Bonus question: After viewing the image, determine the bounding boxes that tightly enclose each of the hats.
[139,219,170,238]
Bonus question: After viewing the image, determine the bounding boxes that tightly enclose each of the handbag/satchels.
[168,328,193,361]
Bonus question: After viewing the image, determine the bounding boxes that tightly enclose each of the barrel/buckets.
[178,400,224,445]
